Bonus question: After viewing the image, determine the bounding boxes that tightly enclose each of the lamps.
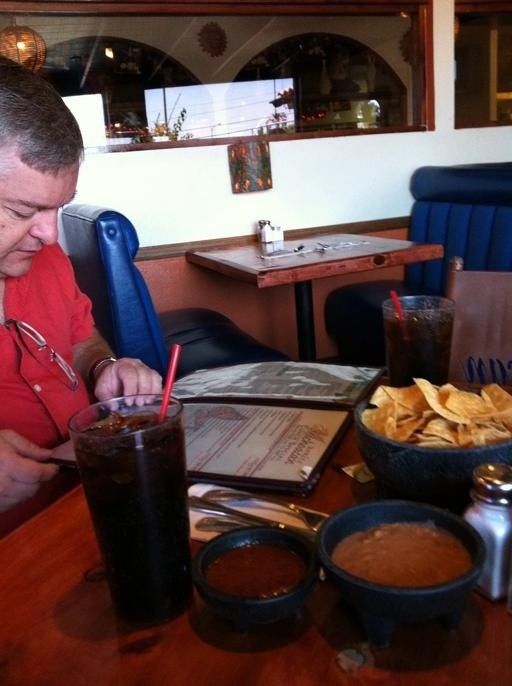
[0,11,46,74]
[227,138,275,196]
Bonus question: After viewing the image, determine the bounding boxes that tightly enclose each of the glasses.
[15,319,78,393]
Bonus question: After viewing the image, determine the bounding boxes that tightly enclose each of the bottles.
[463,460,512,605]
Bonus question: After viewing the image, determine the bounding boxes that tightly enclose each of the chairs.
[444,253,512,387]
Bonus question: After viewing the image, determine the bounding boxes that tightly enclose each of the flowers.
[147,108,193,141]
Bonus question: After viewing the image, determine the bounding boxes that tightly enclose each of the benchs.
[58,202,291,385]
[326,160,512,387]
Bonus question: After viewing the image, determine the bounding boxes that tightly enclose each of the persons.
[0,54,164,517]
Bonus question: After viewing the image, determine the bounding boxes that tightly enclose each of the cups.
[68,394,199,623]
[383,296,454,388]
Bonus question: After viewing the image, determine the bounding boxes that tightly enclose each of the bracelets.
[88,352,121,383]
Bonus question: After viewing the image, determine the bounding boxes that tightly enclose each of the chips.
[360,376,512,448]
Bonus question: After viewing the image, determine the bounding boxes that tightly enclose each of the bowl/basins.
[194,525,317,622]
[350,396,510,493]
[316,501,484,615]
